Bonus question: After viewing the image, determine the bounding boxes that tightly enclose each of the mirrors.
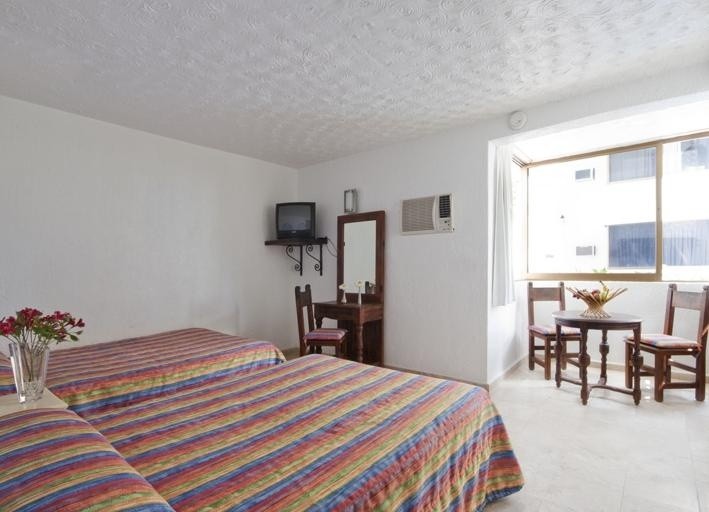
[337,211,384,304]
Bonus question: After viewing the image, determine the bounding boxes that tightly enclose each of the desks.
[314,297,384,367]
[551,310,643,406]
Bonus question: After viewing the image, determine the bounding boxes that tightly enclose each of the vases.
[7,342,50,405]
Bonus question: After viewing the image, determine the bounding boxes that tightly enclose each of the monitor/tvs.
[275,202,316,238]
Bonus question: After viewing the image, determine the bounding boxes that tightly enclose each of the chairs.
[295,284,348,360]
[623,283,709,402]
[528,281,586,380]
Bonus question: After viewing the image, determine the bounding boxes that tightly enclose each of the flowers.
[0,307,85,382]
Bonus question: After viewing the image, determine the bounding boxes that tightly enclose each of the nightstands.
[0,386,70,418]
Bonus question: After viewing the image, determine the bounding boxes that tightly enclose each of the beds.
[1,353,517,511]
[0,327,286,418]
